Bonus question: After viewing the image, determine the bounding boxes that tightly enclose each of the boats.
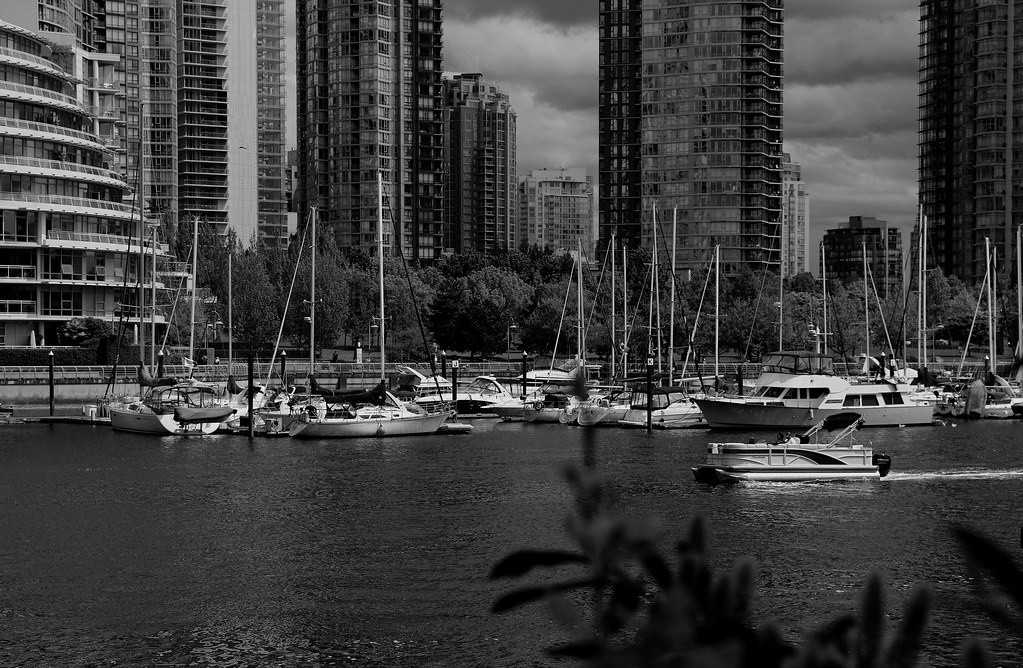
[689,412,891,485]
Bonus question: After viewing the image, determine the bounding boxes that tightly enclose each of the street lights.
[508,317,517,361]
[932,317,944,362]
[206,310,224,348]
[369,319,379,372]
[112,307,125,334]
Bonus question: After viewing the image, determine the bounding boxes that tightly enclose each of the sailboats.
[83,102,1023,438]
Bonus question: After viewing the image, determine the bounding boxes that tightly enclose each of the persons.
[749,437,755,444]
[984,372,995,405]
[215,357,220,365]
[333,351,339,362]
[774,432,791,444]
[722,373,730,393]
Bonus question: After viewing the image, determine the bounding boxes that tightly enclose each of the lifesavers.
[534,400,543,412]
